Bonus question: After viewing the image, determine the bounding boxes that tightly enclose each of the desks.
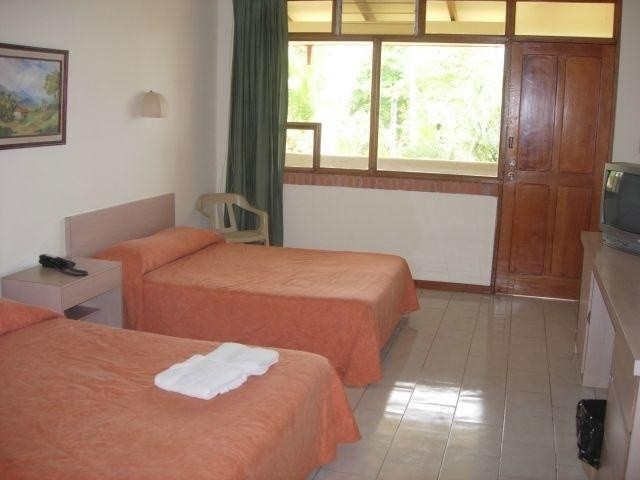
[575,231,640,479]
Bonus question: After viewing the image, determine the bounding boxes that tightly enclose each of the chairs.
[196,193,269,247]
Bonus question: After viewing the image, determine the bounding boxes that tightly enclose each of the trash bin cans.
[576,399,607,480]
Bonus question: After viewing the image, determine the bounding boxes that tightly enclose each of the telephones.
[39,255,75,270]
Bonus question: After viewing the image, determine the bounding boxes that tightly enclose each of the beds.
[0,301,362,480]
[64,194,421,387]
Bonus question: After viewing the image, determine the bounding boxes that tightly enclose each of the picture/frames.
[1,42,69,150]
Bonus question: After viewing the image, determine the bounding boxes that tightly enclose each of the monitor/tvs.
[599,161,640,256]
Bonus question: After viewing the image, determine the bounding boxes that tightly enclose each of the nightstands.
[0,256,123,329]
